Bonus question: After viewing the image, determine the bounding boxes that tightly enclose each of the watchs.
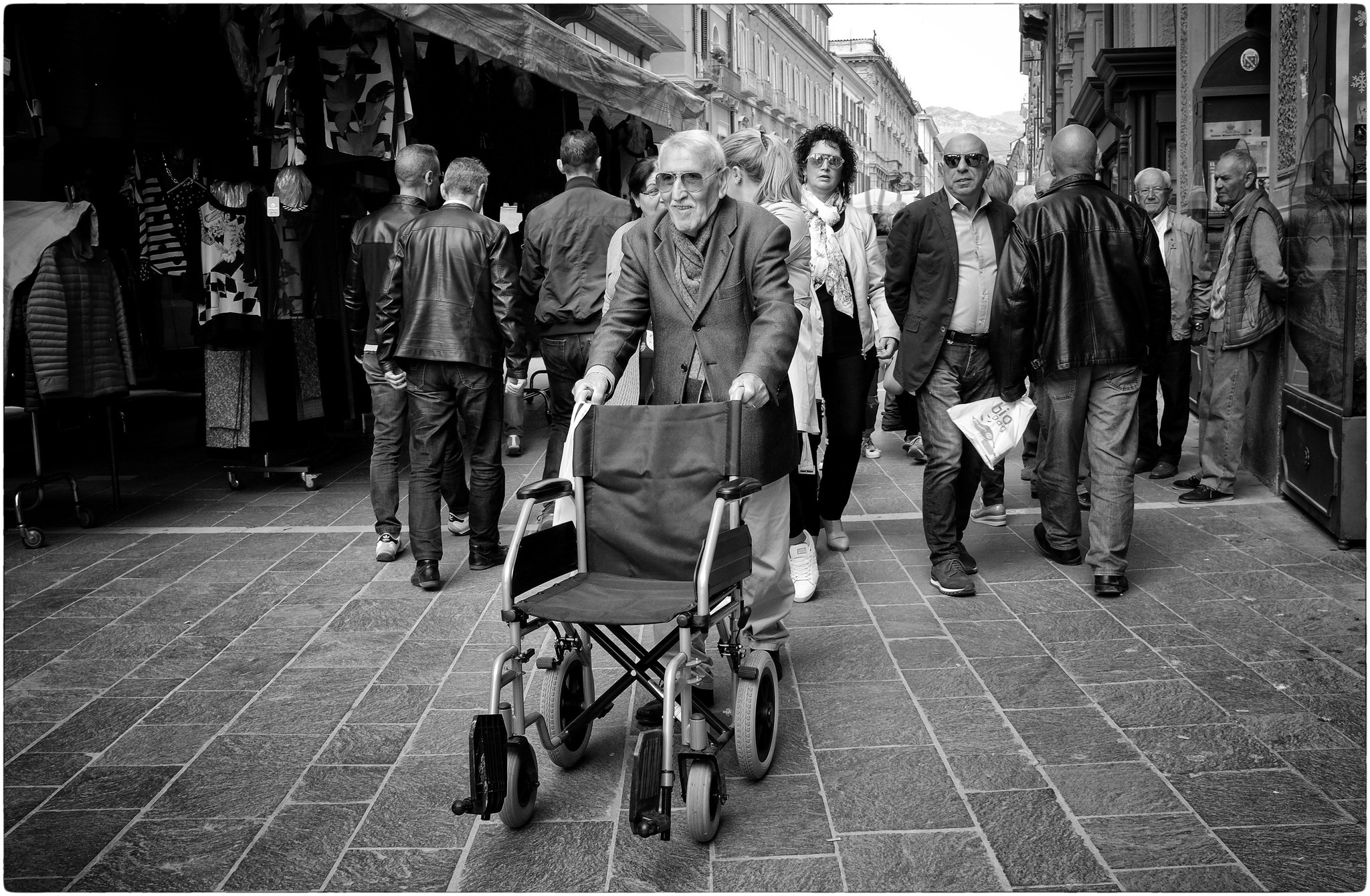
[1194,323,1205,331]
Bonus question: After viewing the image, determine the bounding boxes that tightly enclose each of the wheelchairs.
[447,384,780,847]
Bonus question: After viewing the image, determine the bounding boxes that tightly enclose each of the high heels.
[819,513,849,552]
[970,489,1008,527]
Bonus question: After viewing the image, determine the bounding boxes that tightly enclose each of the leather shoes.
[751,649,783,682]
[635,685,715,723]
[1033,522,1083,566]
[1134,458,1179,480]
[1094,575,1130,594]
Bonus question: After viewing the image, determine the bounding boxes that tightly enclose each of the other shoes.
[1021,464,1035,480]
[468,541,510,570]
[410,559,440,588]
[1079,492,1091,512]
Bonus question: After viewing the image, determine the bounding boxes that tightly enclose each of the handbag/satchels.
[946,394,1037,471]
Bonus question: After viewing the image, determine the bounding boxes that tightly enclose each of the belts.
[945,329,991,347]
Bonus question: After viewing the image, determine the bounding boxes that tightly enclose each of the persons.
[1129,165,1212,479]
[1173,149,1288,505]
[571,129,803,730]
[723,125,820,604]
[346,143,471,562]
[518,132,641,530]
[989,125,1173,597]
[1288,148,1354,405]
[884,133,1017,599]
[794,125,903,609]
[374,155,527,590]
[463,155,1098,509]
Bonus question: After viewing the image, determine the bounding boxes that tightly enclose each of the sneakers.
[1173,475,1234,504]
[505,434,523,456]
[789,529,820,604]
[375,532,404,562]
[862,439,881,458]
[903,433,928,463]
[446,511,470,536]
[929,542,979,597]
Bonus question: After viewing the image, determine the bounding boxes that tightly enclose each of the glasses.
[655,167,723,193]
[422,171,444,182]
[1135,187,1172,196]
[942,153,989,168]
[640,188,662,199]
[804,153,845,170]
